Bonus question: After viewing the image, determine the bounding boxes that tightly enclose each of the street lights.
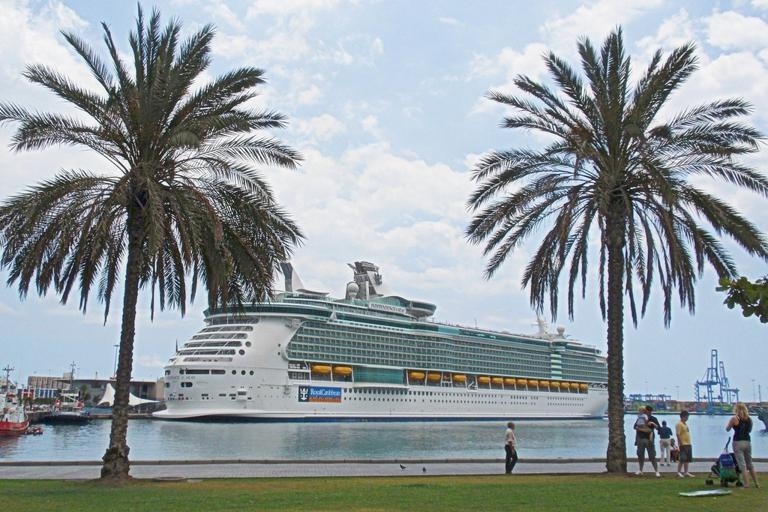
[112,345,120,379]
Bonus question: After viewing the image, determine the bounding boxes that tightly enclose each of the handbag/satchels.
[719,454,738,483]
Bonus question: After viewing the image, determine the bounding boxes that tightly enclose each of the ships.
[150,257,609,422]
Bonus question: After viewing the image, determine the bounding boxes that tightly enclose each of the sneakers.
[635,470,643,475]
[656,471,661,477]
[675,472,684,478]
[684,473,695,477]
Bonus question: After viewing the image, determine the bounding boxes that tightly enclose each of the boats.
[39,390,94,423]
[751,405,768,432]
[0,390,30,437]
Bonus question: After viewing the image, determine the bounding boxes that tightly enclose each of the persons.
[634,406,654,445]
[674,410,694,478]
[633,404,662,477]
[725,401,760,487]
[503,421,519,474]
[0,407,10,421]
[659,420,673,466]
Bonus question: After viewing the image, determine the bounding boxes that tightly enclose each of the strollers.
[705,435,742,488]
